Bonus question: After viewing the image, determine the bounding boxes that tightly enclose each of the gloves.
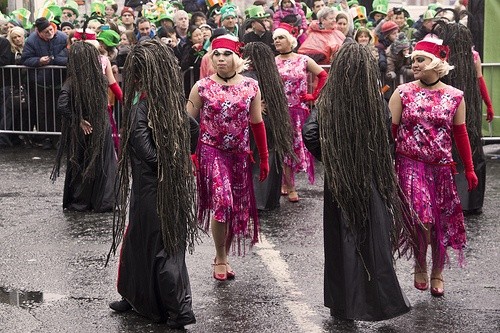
[451,123,479,193]
[478,76,493,123]
[248,119,270,184]
[299,69,328,102]
[109,82,124,105]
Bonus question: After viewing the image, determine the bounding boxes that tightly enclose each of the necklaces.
[217,71,236,83]
[420,78,440,86]
[280,50,292,55]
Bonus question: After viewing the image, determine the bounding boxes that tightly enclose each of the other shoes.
[109,299,134,311]
[430,273,445,295]
[281,190,289,194]
[167,314,196,328]
[288,190,298,202]
[413,263,429,290]
[212,256,235,280]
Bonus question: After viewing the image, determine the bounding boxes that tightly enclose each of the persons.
[0,0,493,297]
[110,38,196,329]
[301,42,412,322]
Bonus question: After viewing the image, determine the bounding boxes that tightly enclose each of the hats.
[96,30,121,47]
[35,17,51,32]
[90,2,107,20]
[369,0,389,20]
[350,5,368,24]
[248,5,270,19]
[155,9,175,24]
[272,22,298,50]
[121,7,136,20]
[421,4,440,20]
[214,4,239,21]
[381,21,398,33]
[205,0,224,19]
[61,0,79,18]
[166,0,185,11]
